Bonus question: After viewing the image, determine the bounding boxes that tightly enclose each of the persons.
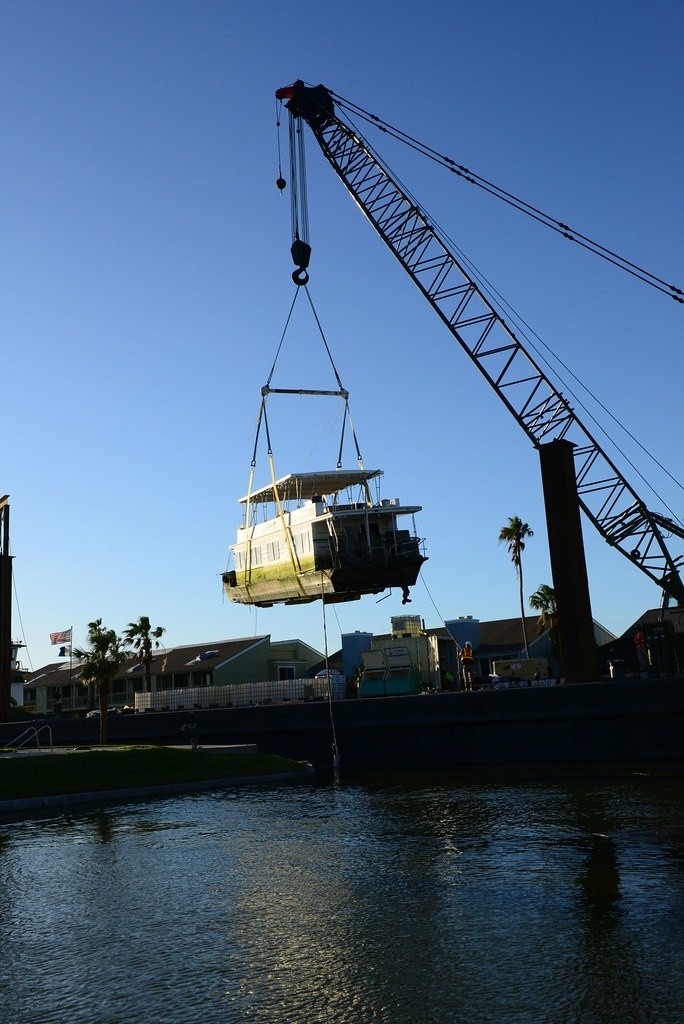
[633,623,648,672]
[459,641,474,691]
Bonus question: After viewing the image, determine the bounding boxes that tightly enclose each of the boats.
[219,468,429,606]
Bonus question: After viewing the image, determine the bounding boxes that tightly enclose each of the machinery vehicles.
[272,76,684,620]
